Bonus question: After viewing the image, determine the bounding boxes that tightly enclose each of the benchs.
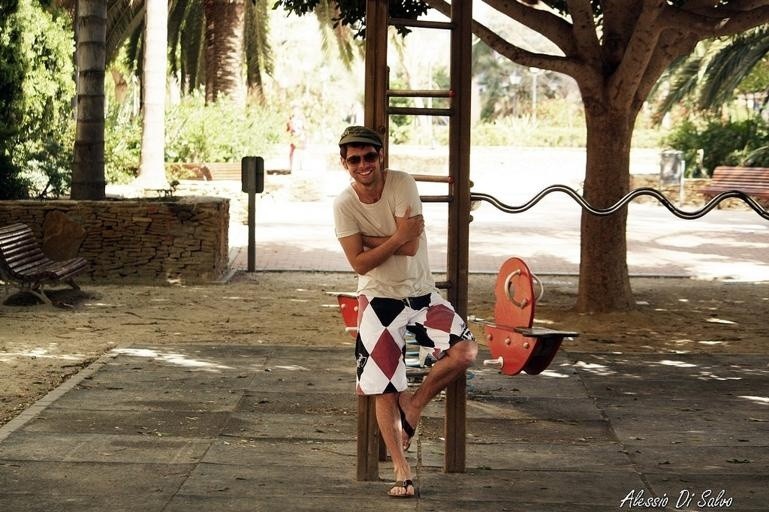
[0,222,90,305]
[692,164,769,210]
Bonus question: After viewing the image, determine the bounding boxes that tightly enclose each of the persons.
[332,126,478,499]
[287,104,304,174]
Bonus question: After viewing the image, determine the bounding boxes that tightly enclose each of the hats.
[338,126,384,148]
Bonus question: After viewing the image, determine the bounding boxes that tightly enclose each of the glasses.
[345,151,379,165]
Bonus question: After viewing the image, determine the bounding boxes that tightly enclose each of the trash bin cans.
[660,149,685,204]
[266,123,295,175]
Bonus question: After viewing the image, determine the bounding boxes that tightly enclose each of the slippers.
[397,393,416,452]
[387,479,415,497]
